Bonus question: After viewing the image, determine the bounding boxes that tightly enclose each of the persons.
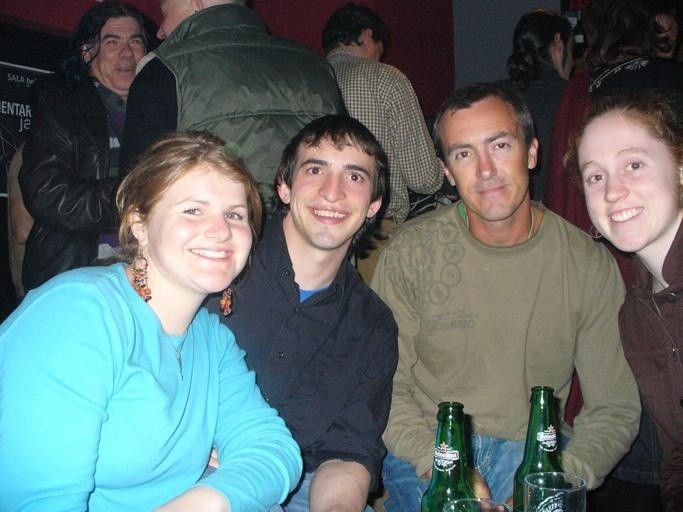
[375,83,642,512]
[496,2,681,511]
[0,1,445,512]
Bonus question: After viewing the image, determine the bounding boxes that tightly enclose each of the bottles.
[420,401,474,511]
[513,385,570,512]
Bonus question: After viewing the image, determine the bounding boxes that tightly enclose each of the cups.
[441,497,512,512]
[523,472,587,512]
